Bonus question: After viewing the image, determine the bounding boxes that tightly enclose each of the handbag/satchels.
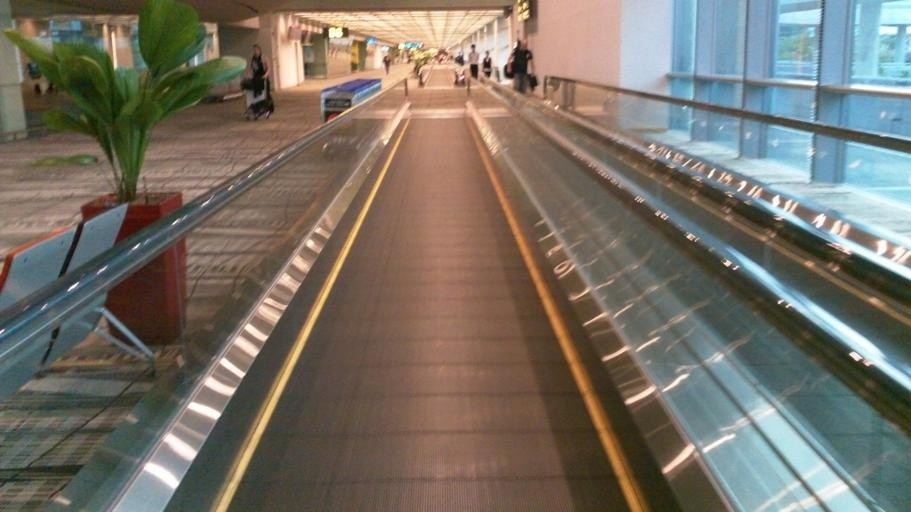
[504,62,514,78]
[241,77,264,91]
[528,73,537,87]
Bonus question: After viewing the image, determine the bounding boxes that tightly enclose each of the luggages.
[246,89,267,120]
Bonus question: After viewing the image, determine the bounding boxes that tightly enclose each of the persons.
[482,50,493,79]
[453,52,464,66]
[382,52,392,76]
[506,38,537,96]
[467,44,480,81]
[244,43,275,116]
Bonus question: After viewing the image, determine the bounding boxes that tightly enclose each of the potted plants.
[3,0,247,346]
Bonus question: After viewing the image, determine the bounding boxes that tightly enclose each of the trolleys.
[239,77,272,121]
